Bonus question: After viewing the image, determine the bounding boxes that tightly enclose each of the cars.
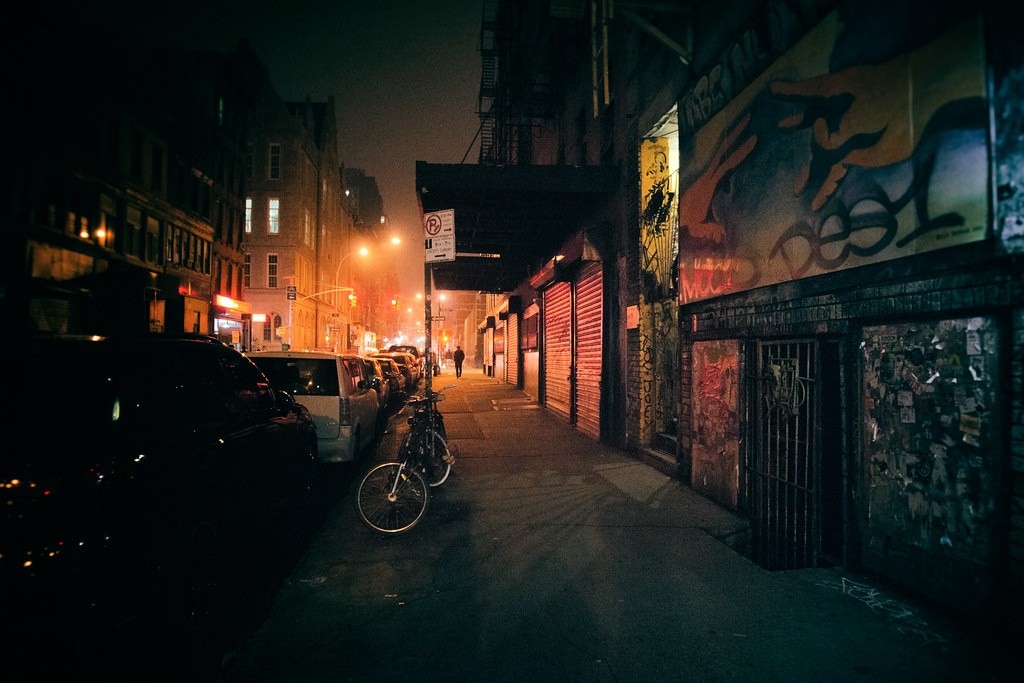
[361,346,424,421]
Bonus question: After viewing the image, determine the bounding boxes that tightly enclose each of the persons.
[453,346,465,379]
[445,349,452,368]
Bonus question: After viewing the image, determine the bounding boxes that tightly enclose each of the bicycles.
[355,391,453,536]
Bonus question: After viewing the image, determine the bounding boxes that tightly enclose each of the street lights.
[329,243,368,353]
[287,288,354,350]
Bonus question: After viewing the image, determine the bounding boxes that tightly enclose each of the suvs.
[242,351,380,466]
[0,329,320,614]
